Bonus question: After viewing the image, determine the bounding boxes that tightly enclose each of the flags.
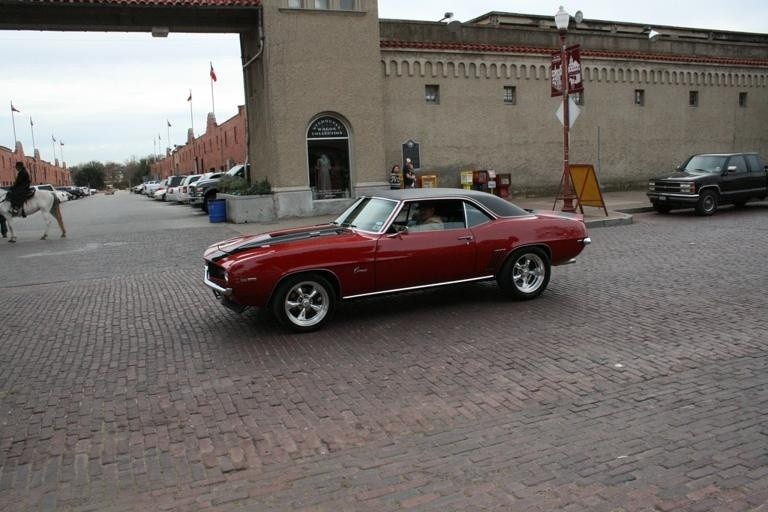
[211,64,217,82]
[187,93,192,102]
[11,105,20,111]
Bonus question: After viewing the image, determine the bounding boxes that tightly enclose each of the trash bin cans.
[208,198,226,223]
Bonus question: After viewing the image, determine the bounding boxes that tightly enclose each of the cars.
[200,189,591,334]
[32,183,95,202]
[645,152,767,216]
[134,164,250,213]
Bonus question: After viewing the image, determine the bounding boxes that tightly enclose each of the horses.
[0,189,67,243]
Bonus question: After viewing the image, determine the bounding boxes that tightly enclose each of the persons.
[387,164,403,190]
[8,159,31,216]
[392,200,446,233]
[315,154,334,199]
[402,158,417,189]
[406,162,417,188]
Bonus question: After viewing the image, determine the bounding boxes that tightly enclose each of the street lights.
[554,6,577,214]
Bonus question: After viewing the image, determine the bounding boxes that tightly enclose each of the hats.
[406,157,412,162]
[414,201,434,211]
[13,161,27,168]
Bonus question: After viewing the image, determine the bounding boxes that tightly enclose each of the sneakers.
[7,206,19,215]
